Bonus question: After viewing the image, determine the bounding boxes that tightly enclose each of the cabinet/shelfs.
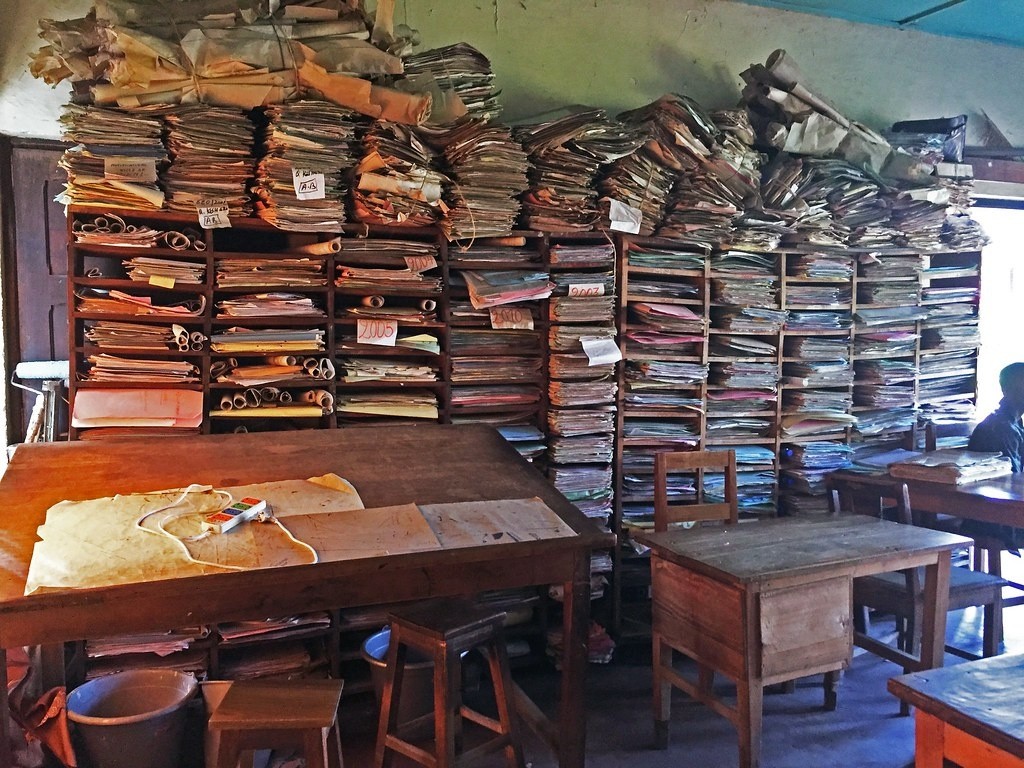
[618,237,980,522]
[66,205,615,677]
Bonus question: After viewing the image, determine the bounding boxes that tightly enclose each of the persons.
[967,362,1024,553]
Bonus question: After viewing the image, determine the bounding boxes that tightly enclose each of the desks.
[886,650,1024,768]
[633,514,974,768]
[0,424,615,768]
[833,451,1024,530]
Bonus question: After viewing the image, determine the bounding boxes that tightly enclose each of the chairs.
[926,423,1024,642]
[652,449,795,700]
[818,470,1009,715]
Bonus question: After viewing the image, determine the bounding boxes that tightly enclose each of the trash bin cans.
[358,627,460,734]
[63,668,199,768]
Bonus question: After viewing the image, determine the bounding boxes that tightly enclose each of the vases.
[64,674,198,768]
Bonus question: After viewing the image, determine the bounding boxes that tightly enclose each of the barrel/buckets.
[67,669,199,768]
[360,630,469,727]
[198,681,271,768]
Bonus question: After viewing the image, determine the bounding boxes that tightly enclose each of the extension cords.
[202,497,266,533]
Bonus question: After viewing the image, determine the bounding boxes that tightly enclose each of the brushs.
[9,359,70,445]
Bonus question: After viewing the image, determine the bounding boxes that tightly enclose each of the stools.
[372,607,522,768]
[206,676,345,768]
